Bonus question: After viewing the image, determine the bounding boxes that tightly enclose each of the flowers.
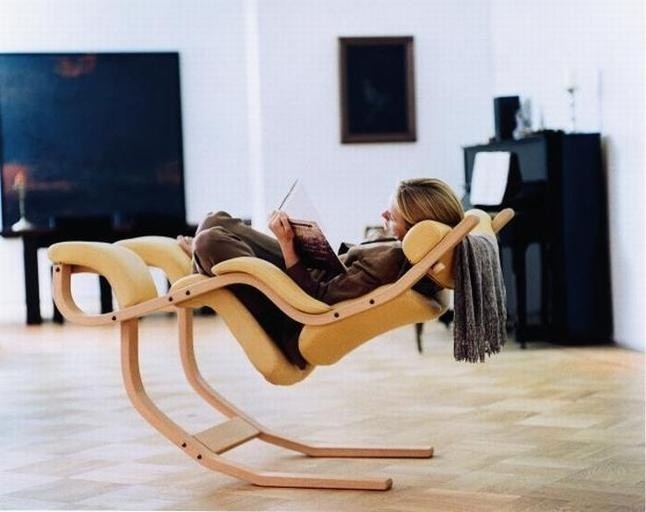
[10,167,28,216]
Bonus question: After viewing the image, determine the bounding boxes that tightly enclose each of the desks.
[0,229,217,325]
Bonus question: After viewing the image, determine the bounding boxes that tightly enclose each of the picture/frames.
[336,35,420,147]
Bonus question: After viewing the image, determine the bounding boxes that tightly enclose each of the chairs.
[130,209,184,320]
[46,208,516,495]
[48,210,114,322]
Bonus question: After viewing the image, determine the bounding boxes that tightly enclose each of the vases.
[10,216,35,231]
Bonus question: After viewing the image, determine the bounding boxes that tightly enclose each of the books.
[271,177,349,276]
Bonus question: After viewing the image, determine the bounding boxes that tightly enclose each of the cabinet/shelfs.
[463,128,616,348]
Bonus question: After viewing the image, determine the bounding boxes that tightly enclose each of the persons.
[174,177,463,369]
